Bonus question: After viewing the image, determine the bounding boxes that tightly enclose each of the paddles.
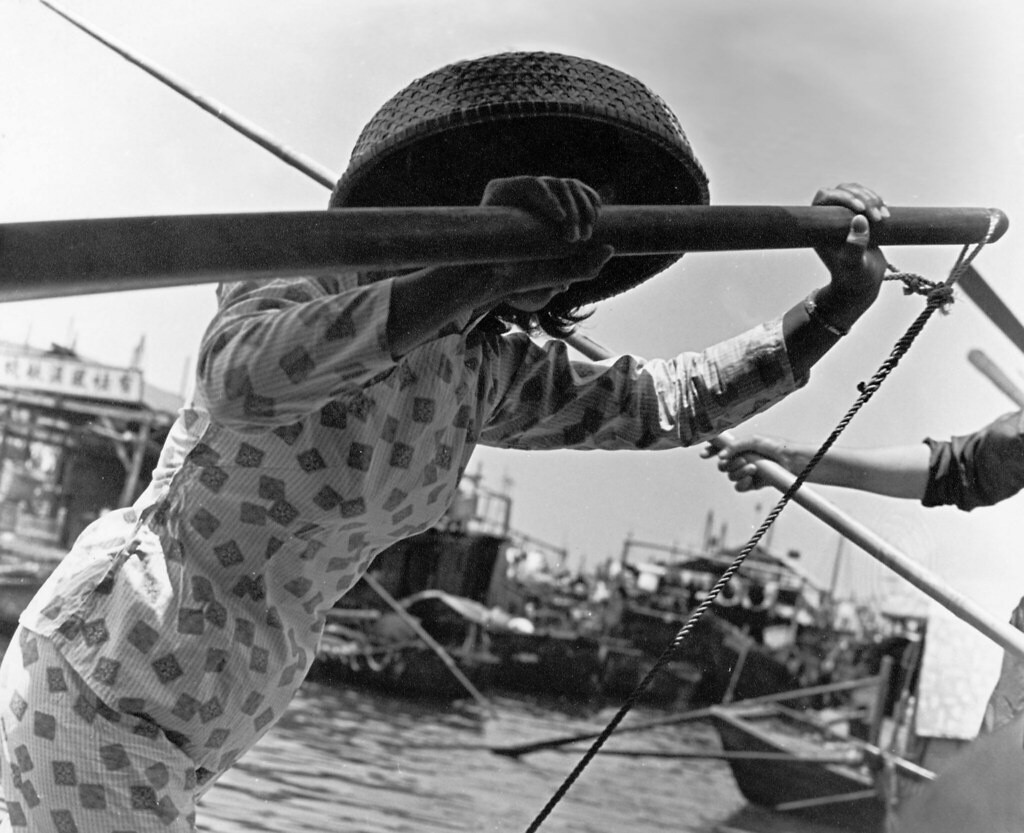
[0,202,1012,310]
[775,702,937,781]
[362,571,489,708]
[549,744,865,767]
[492,675,883,761]
[33,0,1024,665]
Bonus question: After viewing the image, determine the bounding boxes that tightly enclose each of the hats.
[324,50,710,310]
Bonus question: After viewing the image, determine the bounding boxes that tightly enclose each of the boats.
[707,703,972,823]
[0,343,929,708]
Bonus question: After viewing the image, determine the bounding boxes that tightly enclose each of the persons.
[0,53,891,833]
[701,403,1024,743]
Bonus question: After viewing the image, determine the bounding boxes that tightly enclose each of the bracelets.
[804,294,847,336]
